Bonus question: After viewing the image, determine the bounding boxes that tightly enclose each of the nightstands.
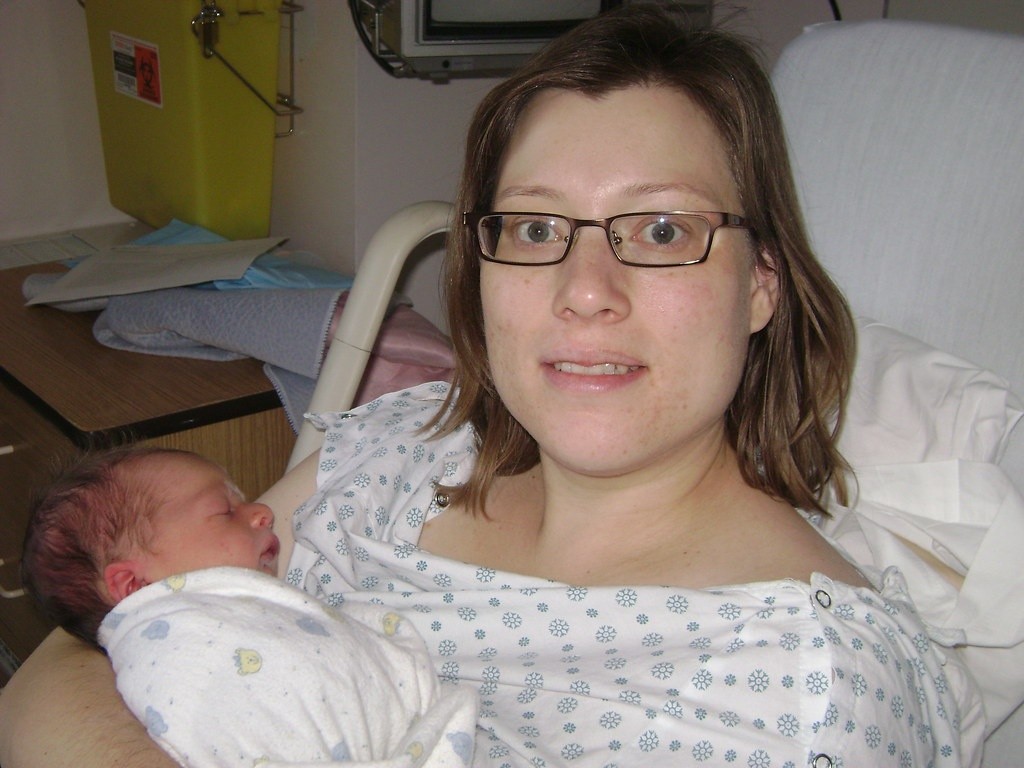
[0,260,296,681]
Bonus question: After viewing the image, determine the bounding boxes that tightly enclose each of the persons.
[24,444,477,768]
[1,17,962,768]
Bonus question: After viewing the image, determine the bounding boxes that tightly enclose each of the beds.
[290,205,1024,768]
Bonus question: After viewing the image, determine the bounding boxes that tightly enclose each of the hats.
[140,56,154,71]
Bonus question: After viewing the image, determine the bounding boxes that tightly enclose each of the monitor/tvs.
[362,0,623,74]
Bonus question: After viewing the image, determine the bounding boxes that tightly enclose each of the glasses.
[463,200,751,268]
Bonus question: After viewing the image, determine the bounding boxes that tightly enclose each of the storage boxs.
[85,0,283,241]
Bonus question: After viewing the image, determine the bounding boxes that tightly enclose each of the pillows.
[768,17,1024,401]
